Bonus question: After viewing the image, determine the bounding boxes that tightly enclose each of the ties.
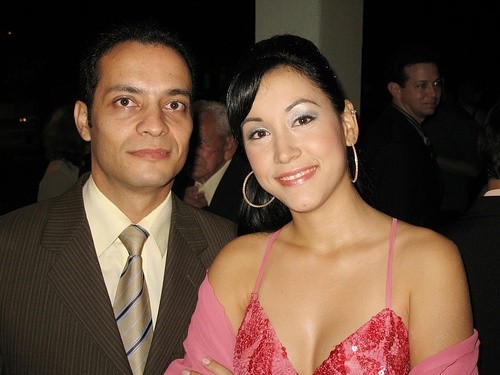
[113,224,154,375]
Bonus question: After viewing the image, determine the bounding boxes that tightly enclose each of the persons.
[174,100,292,237]
[157,35,482,375]
[0,25,239,374]
[36,104,92,203]
[353,42,485,232]
[416,78,500,375]
